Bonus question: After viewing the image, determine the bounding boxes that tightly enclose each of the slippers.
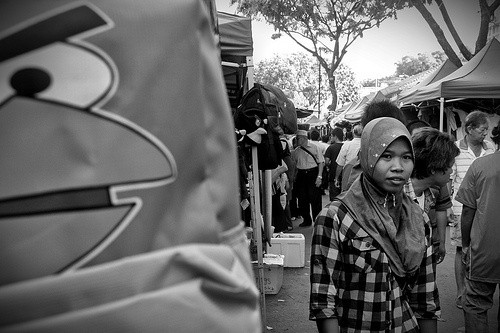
[455,295,463,309]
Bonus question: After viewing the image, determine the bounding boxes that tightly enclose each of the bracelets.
[317,176,323,179]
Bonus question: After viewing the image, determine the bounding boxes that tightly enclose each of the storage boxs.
[251,254,286,294]
[270,233,306,268]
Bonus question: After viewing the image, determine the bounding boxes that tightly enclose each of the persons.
[454,119,500,333]
[345,100,417,212]
[308,117,442,333]
[444,109,497,311]
[267,120,381,233]
[399,126,456,333]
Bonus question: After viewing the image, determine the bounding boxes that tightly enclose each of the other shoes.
[299,221,312,227]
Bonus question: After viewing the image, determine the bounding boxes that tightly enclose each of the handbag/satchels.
[321,167,329,189]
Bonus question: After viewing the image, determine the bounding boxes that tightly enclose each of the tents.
[318,59,461,127]
[207,3,279,333]
[412,37,500,163]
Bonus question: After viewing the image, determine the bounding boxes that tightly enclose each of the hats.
[297,130,307,136]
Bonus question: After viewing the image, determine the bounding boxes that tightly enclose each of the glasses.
[297,136,303,140]
